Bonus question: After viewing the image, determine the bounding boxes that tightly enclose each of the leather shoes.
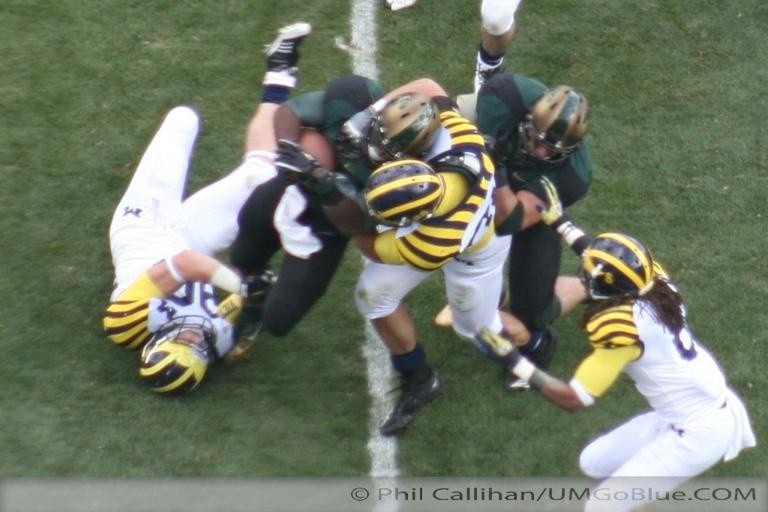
[477,328,522,372]
[239,266,272,299]
[274,139,321,178]
[535,175,568,235]
[335,106,375,163]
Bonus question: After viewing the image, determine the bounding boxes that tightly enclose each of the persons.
[104,0,591,436]
[475,174,757,512]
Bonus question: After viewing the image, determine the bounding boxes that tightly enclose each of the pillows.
[366,93,443,163]
[364,159,444,227]
[139,315,217,398]
[579,232,655,301]
[524,85,590,166]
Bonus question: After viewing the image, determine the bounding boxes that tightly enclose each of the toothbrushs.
[298,126,336,179]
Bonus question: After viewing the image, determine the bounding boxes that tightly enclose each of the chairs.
[506,331,556,391]
[265,22,311,71]
[225,340,255,361]
[380,370,443,437]
